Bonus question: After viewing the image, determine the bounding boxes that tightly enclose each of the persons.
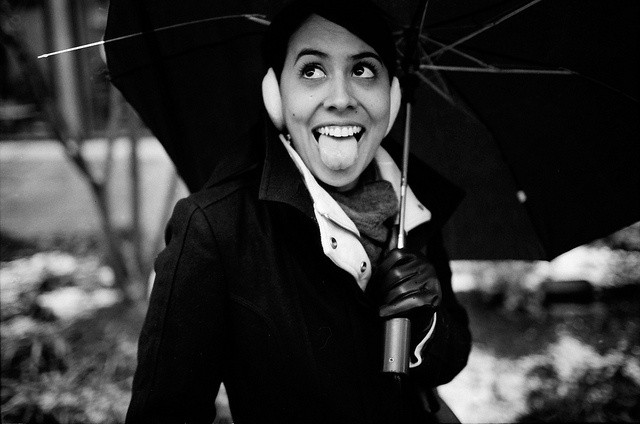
[126,1,471,424]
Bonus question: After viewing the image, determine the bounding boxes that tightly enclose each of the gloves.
[371,248,442,334]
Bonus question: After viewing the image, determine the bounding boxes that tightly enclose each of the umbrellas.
[104,0,638,374]
